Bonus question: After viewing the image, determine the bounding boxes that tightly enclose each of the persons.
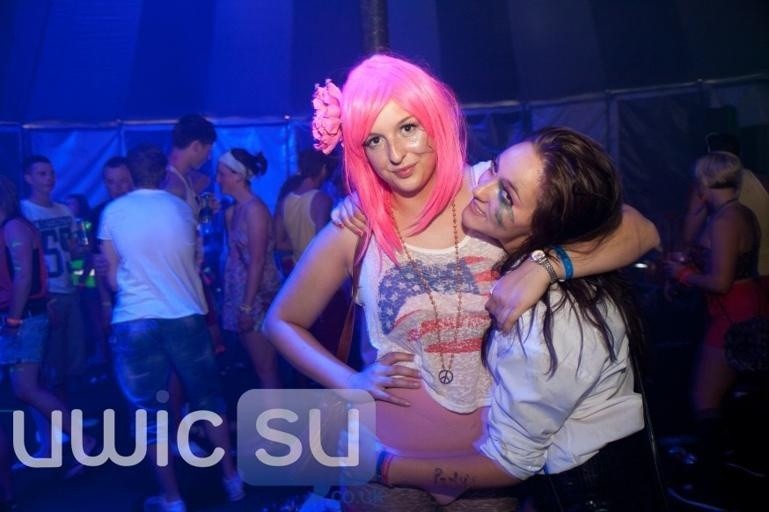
[262,51,662,510]
[339,125,663,512]
[676,127,769,295]
[93,136,251,512]
[61,113,334,465]
[17,154,81,403]
[647,147,766,471]
[0,171,101,477]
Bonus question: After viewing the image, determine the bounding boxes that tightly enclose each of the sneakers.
[143,497,186,512]
[10,440,46,473]
[222,473,247,501]
[65,431,99,475]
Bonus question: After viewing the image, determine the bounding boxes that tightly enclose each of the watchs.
[527,245,559,284]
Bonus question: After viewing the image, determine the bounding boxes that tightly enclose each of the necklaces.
[385,196,466,385]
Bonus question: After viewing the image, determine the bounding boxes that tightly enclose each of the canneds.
[72,218,89,247]
[201,192,215,224]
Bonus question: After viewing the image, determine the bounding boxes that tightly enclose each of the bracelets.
[679,266,694,285]
[375,446,399,491]
[555,246,575,282]
